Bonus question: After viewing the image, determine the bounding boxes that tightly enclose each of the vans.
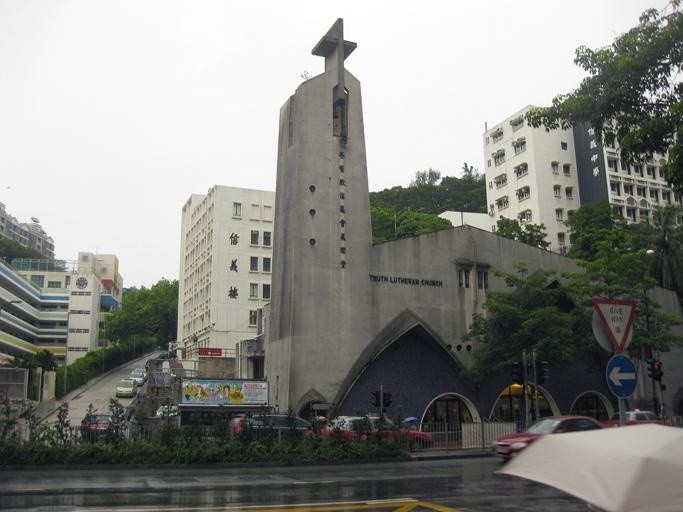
[115,378,138,399]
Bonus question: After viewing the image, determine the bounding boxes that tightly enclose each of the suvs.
[320,417,435,453]
[227,412,318,444]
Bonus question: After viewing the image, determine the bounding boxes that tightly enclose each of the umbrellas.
[493,421,683,511]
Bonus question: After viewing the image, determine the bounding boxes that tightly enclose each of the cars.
[128,367,148,387]
[80,413,116,435]
[603,409,674,428]
[490,415,609,460]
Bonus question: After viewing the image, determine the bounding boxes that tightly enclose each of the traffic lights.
[646,356,664,381]
